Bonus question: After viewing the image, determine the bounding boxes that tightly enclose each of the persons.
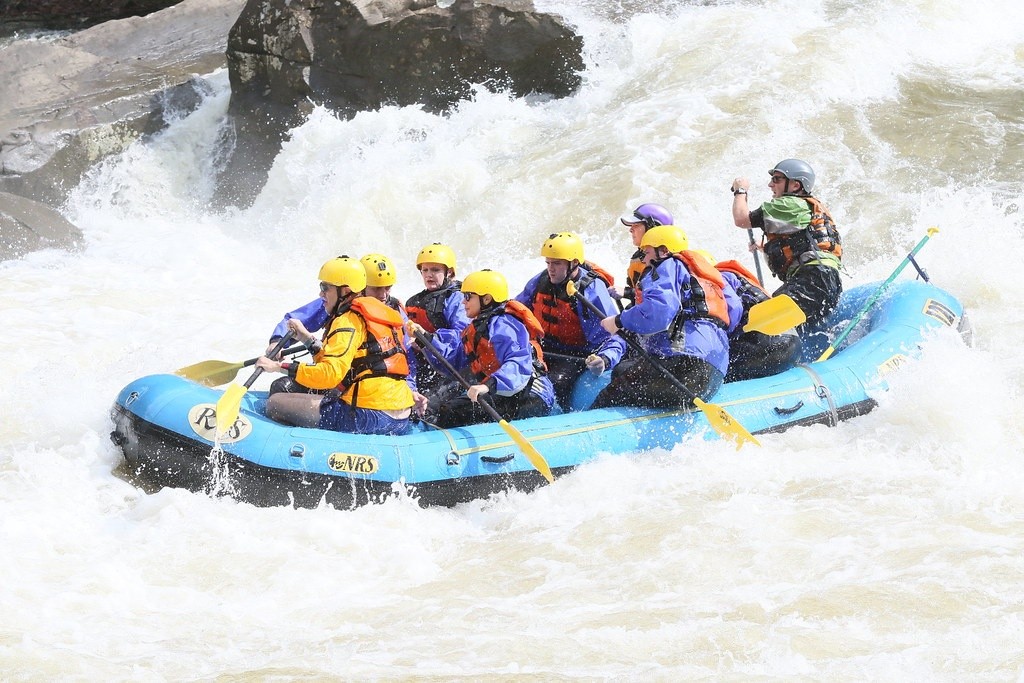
[732,159,843,331]
[514,233,626,404]
[607,203,674,310]
[267,254,428,418]
[405,243,471,396]
[588,225,730,408]
[694,249,802,383]
[405,269,555,429]
[255,255,416,435]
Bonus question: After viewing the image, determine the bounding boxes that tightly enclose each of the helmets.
[634,203,673,227]
[541,232,585,265]
[318,256,368,294]
[460,269,509,304]
[640,225,687,256]
[768,160,815,194]
[415,243,456,277]
[360,254,396,288]
[633,202,672,229]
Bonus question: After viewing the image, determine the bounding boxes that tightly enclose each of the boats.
[107,277,969,506]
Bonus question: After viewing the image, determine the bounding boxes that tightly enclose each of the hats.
[620,215,647,227]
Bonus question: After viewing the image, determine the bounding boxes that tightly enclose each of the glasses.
[320,282,343,293]
[463,292,478,301]
[770,176,787,183]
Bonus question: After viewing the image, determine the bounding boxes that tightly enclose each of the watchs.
[734,188,748,196]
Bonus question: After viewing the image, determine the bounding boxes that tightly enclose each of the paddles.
[729,293,808,342]
[404,322,555,487]
[174,339,323,389]
[613,297,624,313]
[742,200,767,290]
[215,325,296,435]
[566,279,763,453]
[543,350,597,366]
[811,225,941,365]
